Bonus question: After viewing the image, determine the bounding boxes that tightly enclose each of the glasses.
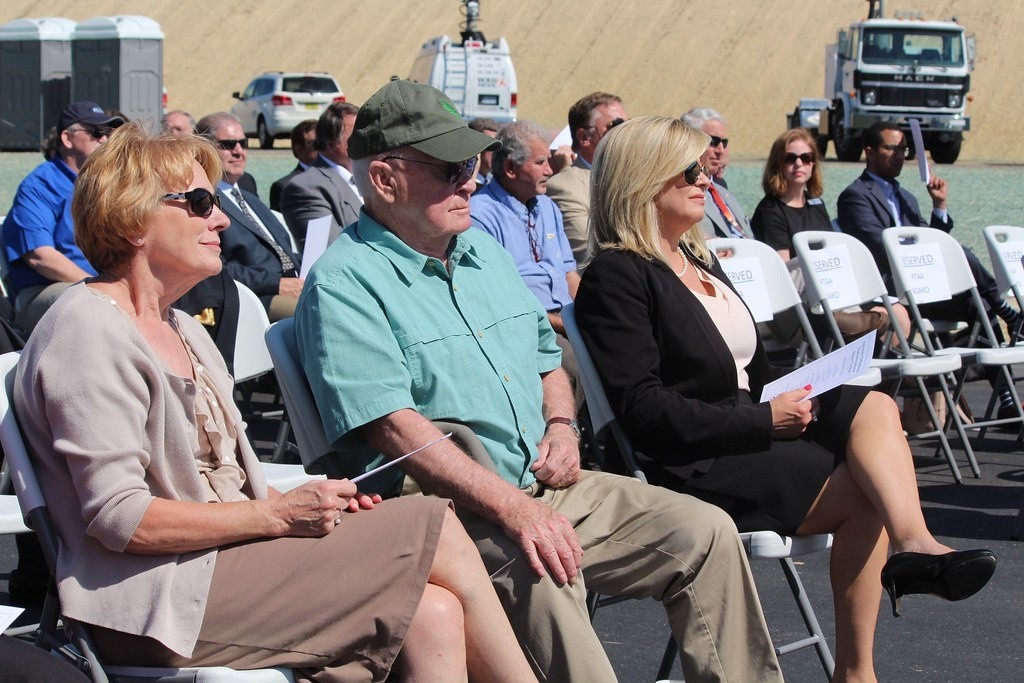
[162,189,221,220]
[708,136,729,148]
[217,138,249,150]
[786,153,813,163]
[74,127,111,138]
[384,156,477,184]
[884,145,910,156]
[683,162,708,185]
[588,118,624,128]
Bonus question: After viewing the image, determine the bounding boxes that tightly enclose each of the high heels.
[881,549,996,618]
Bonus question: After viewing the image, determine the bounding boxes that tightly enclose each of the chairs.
[922,49,940,60]
[0,217,1024,683]
[864,44,881,57]
[299,84,310,90]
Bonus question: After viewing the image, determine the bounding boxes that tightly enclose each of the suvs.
[230,70,346,148]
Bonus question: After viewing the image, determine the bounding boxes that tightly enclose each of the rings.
[811,409,818,422]
[334,508,343,524]
[558,481,562,487]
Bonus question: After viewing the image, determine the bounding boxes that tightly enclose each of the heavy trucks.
[785,0,977,163]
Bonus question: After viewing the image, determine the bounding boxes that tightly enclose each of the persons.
[11,122,540,683]
[749,127,911,359]
[835,120,1024,430]
[571,115,998,683]
[0,90,762,385]
[295,74,787,683]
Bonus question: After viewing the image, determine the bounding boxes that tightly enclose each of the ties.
[709,183,749,239]
[231,187,295,270]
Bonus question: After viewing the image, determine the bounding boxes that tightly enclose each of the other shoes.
[997,403,1023,431]
[1006,310,1024,342]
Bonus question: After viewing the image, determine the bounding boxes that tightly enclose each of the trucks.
[407,0,519,126]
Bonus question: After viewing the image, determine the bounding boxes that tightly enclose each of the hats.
[347,75,504,162]
[57,100,125,133]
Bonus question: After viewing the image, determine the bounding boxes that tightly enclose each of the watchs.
[545,417,581,437]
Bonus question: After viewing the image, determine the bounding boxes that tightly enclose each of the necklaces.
[675,245,688,278]
